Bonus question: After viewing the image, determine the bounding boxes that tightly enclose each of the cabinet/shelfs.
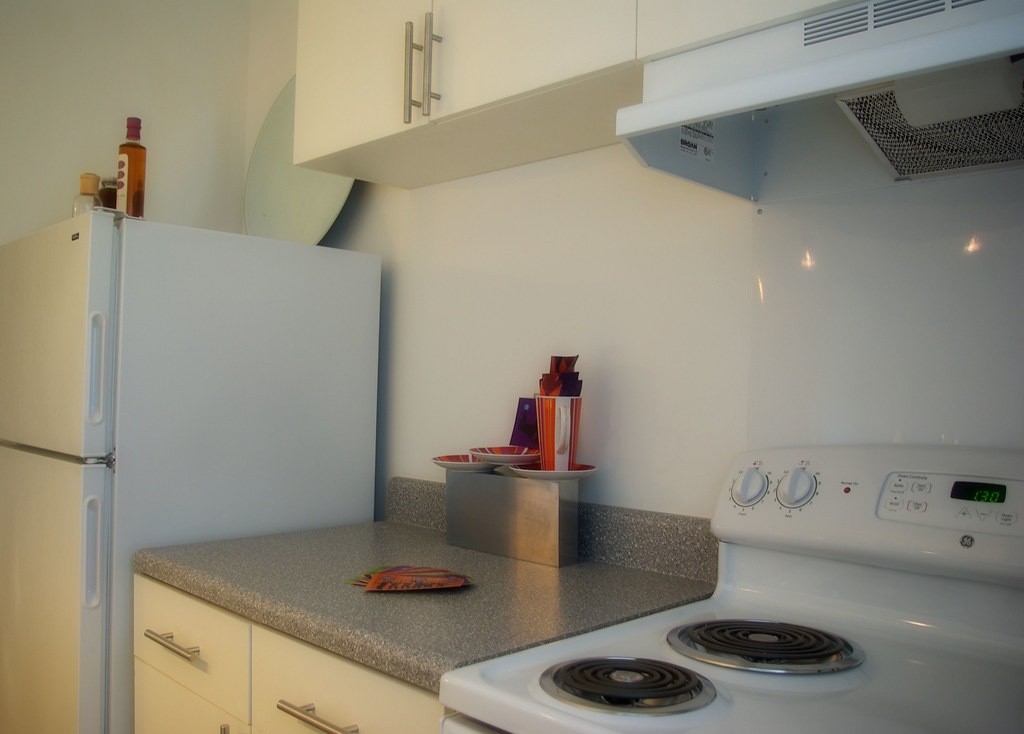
[291,1,868,191]
[132,573,454,734]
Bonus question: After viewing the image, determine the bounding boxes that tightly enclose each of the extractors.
[609,0,1024,204]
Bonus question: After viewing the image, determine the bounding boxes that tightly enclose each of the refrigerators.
[0,212,381,734]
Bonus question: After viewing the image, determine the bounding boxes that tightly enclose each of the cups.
[534,394,583,471]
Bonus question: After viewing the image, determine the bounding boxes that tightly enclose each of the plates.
[507,463,599,479]
[431,455,502,472]
[468,447,540,465]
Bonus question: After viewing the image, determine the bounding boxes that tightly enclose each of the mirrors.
[242,72,355,245]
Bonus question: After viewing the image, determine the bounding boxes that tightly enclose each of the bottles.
[71,117,147,219]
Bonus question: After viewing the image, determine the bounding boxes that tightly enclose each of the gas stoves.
[439,444,1024,734]
